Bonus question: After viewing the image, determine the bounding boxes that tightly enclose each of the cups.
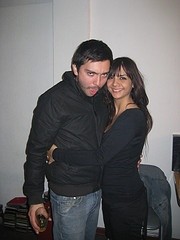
[34,207,49,231]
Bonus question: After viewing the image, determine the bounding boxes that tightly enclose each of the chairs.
[137,162,172,240]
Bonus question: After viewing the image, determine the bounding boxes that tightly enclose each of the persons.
[47,56,154,240]
[22,39,116,240]
